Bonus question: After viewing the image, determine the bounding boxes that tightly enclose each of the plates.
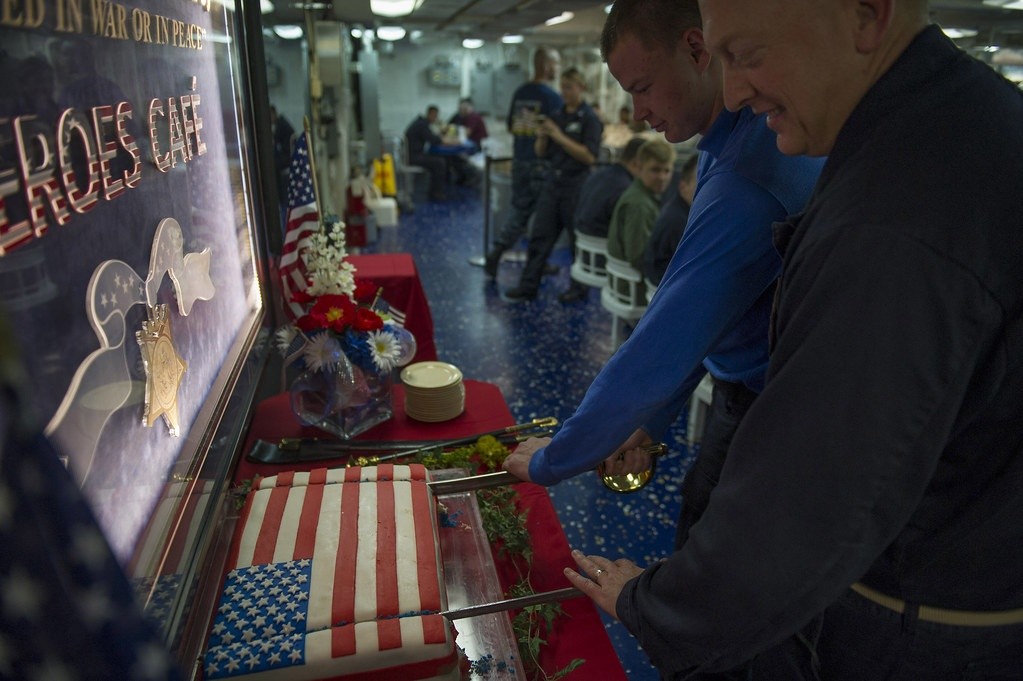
[399,360,466,422]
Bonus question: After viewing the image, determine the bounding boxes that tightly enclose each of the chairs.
[571,228,714,447]
[393,137,424,199]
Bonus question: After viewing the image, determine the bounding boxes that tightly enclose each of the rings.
[595,568,606,583]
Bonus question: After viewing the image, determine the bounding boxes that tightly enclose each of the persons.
[407,98,487,192]
[573,139,699,335]
[564,0,1023,680]
[502,0,828,553]
[271,106,296,195]
[617,106,651,133]
[484,45,604,303]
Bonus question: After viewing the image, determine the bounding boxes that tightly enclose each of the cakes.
[202,462,458,681]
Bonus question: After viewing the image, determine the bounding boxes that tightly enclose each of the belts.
[849,580,1023,627]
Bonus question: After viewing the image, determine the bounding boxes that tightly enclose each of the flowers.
[275,222,400,374]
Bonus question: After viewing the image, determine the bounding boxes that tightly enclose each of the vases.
[294,375,391,439]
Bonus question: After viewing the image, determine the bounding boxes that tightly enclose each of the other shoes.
[558,285,590,302]
[500,286,527,302]
[483,251,500,279]
[539,263,559,276]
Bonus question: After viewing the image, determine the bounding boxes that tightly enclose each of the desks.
[340,255,439,364]
[207,380,624,681]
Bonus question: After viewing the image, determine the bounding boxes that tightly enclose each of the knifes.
[426,441,668,495]
[438,587,584,620]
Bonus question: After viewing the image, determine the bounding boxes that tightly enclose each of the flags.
[279,136,319,324]
